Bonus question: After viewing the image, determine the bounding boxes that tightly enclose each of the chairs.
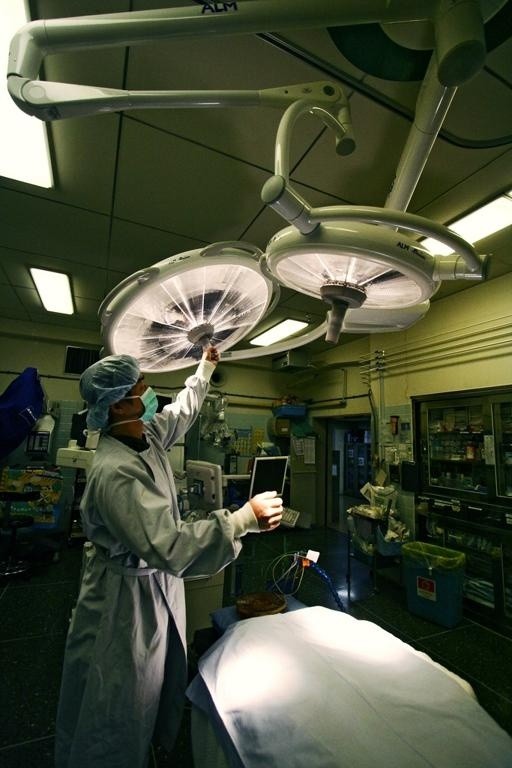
[0,492,41,577]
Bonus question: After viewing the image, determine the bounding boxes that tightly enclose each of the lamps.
[0,0,512,378]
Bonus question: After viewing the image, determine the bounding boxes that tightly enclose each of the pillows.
[209,594,307,636]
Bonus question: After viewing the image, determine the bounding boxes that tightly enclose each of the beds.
[186,605,512,768]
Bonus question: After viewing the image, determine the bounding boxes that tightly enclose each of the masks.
[120,386,159,425]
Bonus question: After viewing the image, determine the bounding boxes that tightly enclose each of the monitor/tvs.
[177,459,223,514]
[249,456,288,501]
[63,345,100,375]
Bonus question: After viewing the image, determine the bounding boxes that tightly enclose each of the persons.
[53,337,286,767]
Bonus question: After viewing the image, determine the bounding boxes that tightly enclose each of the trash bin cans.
[402,541,466,628]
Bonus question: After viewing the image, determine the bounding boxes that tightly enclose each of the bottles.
[465,441,474,461]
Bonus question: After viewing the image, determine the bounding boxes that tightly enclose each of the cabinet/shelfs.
[410,384,512,638]
